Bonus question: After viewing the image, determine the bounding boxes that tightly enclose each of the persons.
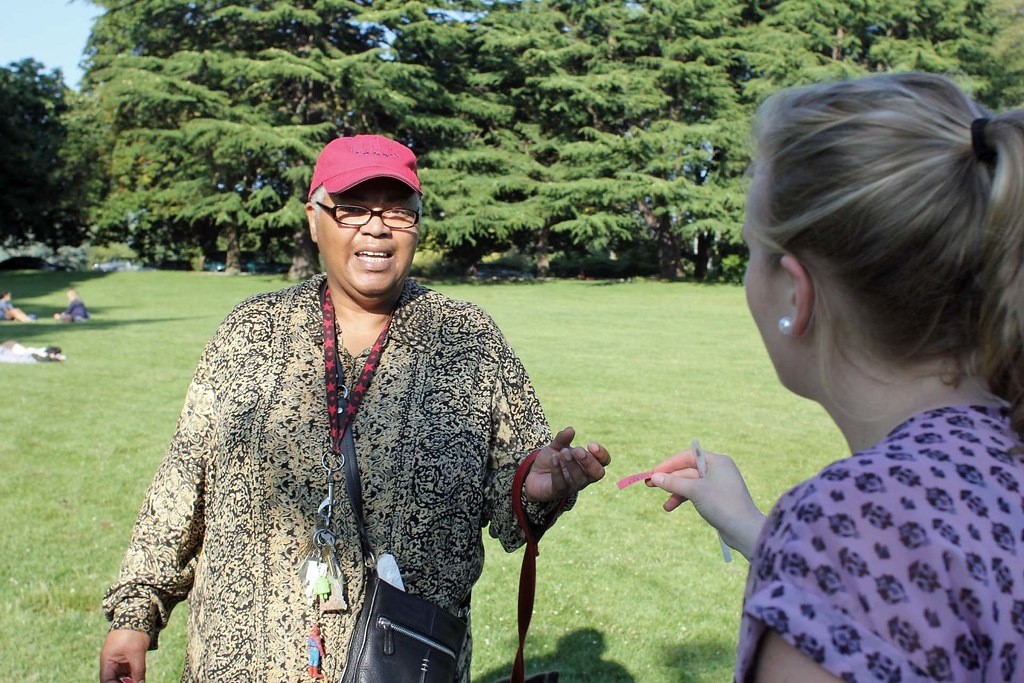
[99,132,612,683]
[641,69,1023,683]
[0,340,68,362]
[0,288,37,323]
[307,625,325,679]
[53,289,89,322]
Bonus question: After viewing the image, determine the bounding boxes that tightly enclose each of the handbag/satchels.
[342,576,467,683]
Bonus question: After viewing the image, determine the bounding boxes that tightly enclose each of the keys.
[297,523,338,579]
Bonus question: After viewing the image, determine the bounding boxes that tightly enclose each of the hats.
[307,133,423,201]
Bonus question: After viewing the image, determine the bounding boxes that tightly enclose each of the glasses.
[316,200,420,230]
